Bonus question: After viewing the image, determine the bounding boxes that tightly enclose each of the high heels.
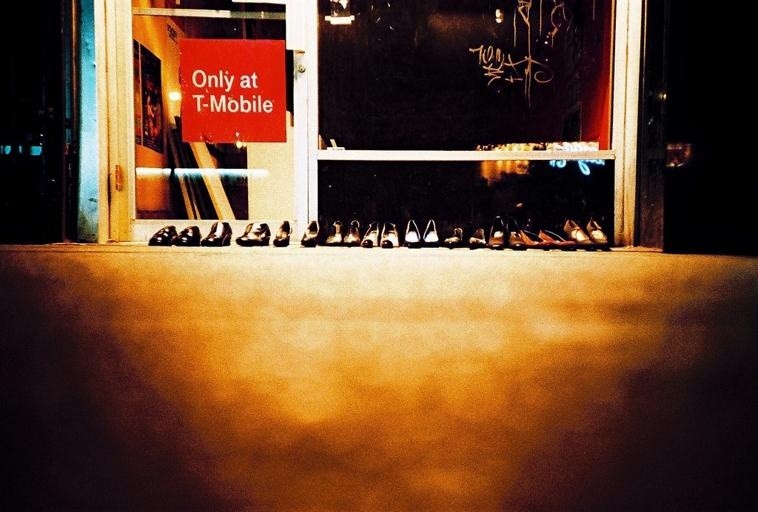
[488,218,506,248]
[506,219,524,249]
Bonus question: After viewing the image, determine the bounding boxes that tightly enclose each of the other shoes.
[587,221,608,248]
[324,221,344,246]
[422,220,441,246]
[273,220,293,247]
[444,225,465,249]
[519,229,549,247]
[361,221,380,247]
[469,227,486,247]
[380,223,400,247]
[405,220,422,247]
[301,220,320,247]
[564,219,594,249]
[538,229,577,248]
[343,220,361,247]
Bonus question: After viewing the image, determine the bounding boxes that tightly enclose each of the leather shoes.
[236,223,270,247]
[174,225,202,246]
[149,226,177,246]
